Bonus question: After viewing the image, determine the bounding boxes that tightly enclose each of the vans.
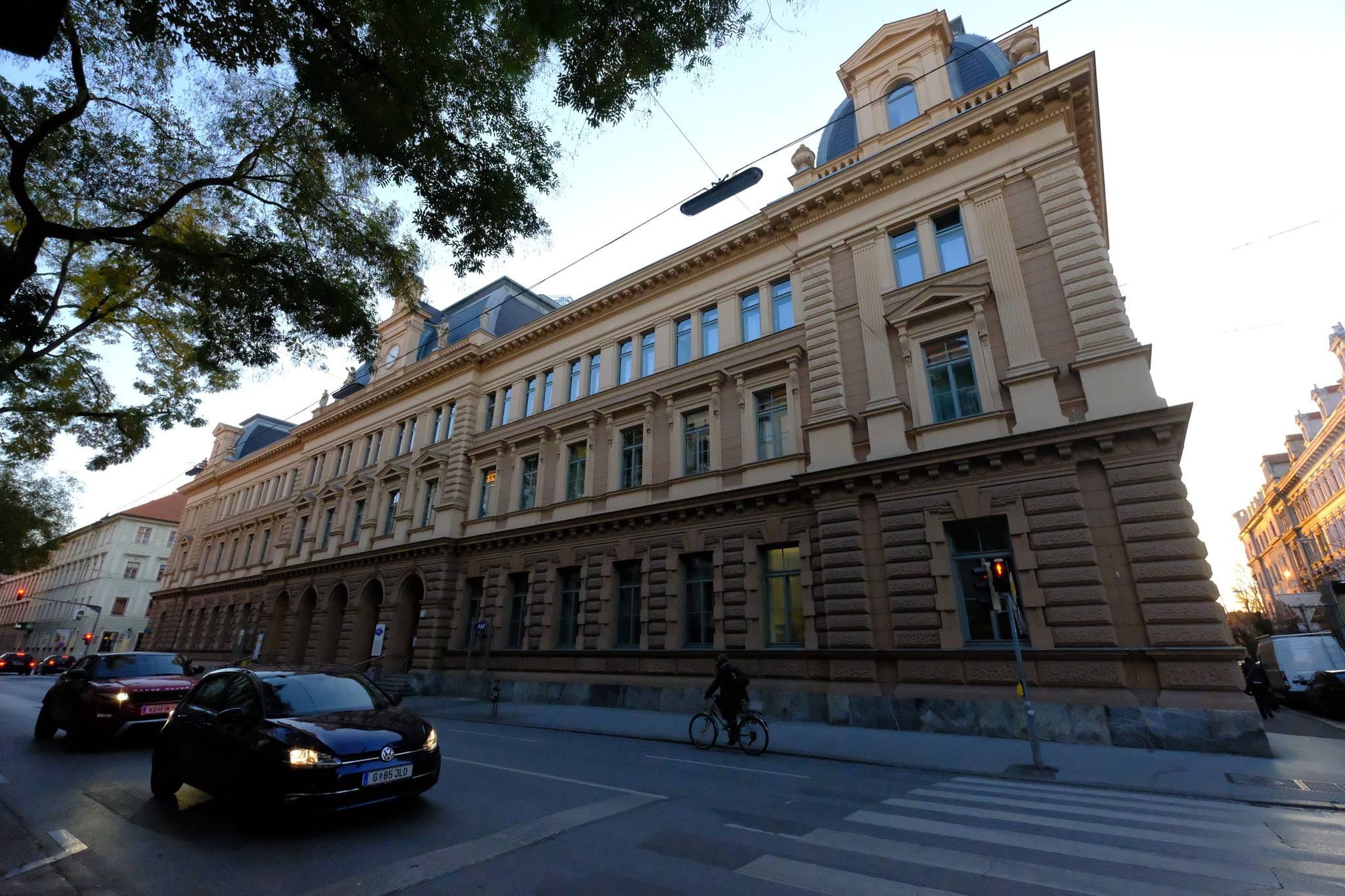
[1257,630,1345,708]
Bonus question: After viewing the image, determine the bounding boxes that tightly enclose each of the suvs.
[0,651,36,675]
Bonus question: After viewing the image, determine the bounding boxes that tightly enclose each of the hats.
[716,654,729,664]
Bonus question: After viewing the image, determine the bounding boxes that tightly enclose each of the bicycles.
[689,694,770,756]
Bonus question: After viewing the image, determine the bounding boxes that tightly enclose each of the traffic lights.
[989,561,1012,599]
[16,589,26,601]
[969,565,994,612]
[118,634,124,642]
[82,633,93,645]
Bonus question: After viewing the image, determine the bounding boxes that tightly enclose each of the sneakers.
[726,736,738,746]
[722,719,734,731]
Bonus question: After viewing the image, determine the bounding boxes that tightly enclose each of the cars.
[150,662,441,821]
[35,652,206,745]
[33,655,77,675]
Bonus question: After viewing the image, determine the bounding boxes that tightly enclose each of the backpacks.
[719,666,750,688]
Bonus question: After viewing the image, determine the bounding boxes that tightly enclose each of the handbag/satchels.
[1266,689,1280,713]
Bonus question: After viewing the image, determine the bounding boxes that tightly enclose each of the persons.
[703,653,750,745]
[1241,656,1276,719]
